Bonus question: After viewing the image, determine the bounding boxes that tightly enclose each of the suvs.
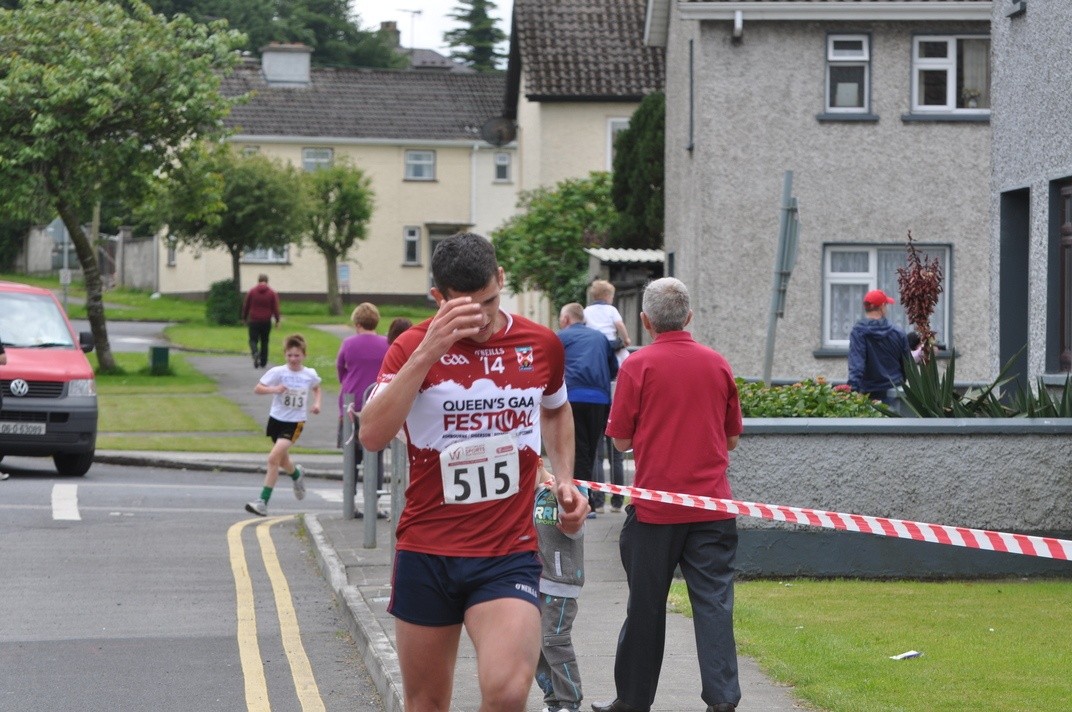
[0,280,98,477]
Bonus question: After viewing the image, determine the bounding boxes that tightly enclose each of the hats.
[864,290,895,309]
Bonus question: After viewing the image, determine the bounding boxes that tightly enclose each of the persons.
[333,279,637,519]
[359,229,592,711]
[244,333,325,517]
[845,291,911,396]
[535,454,589,711]
[589,277,742,712]
[239,274,282,369]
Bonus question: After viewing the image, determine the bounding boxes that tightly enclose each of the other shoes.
[254,353,260,368]
[595,506,605,513]
[591,702,616,712]
[609,505,622,512]
[378,509,389,518]
[293,465,306,500]
[354,511,364,518]
[245,500,268,516]
